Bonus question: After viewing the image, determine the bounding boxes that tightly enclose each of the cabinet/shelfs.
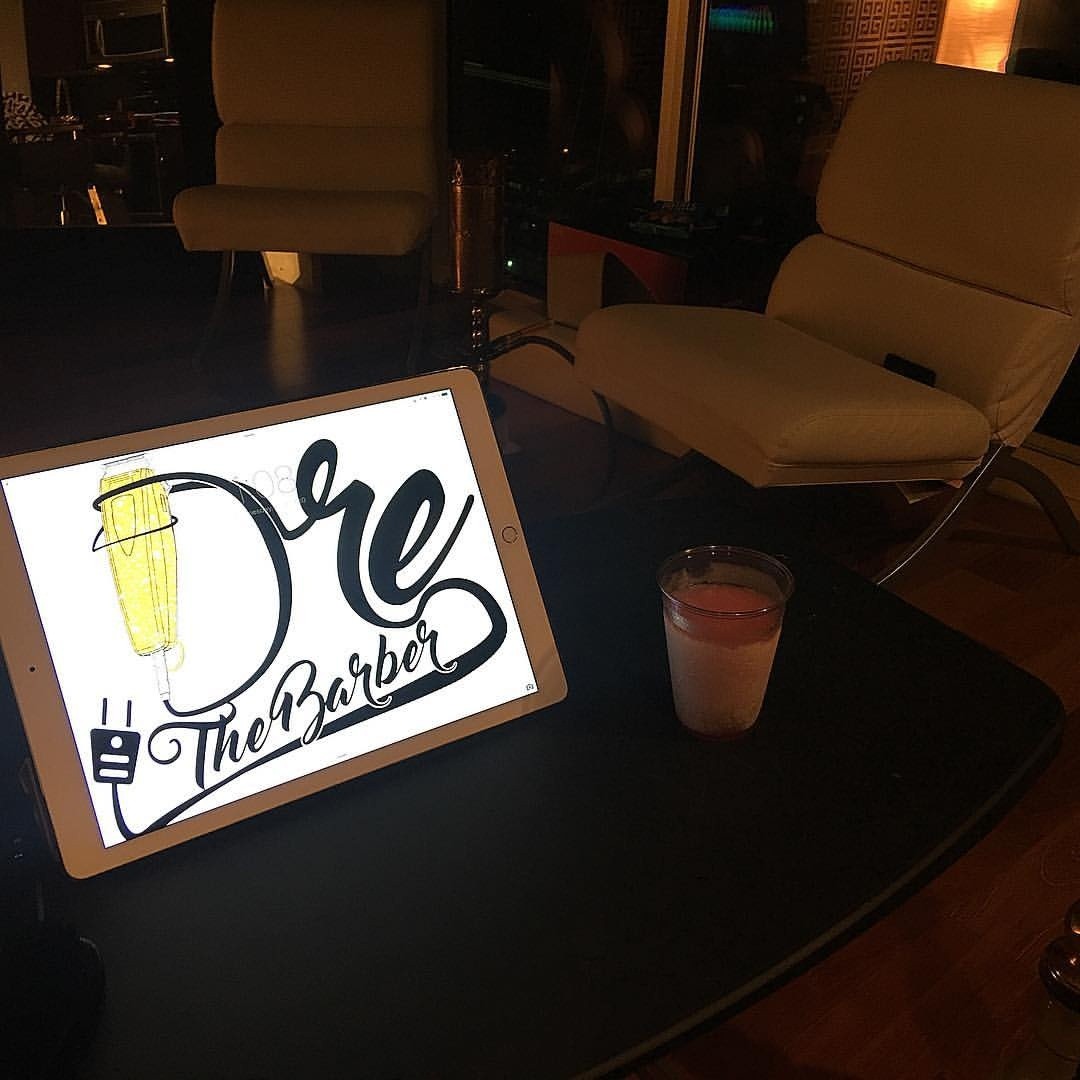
[1,124,181,231]
[546,217,683,331]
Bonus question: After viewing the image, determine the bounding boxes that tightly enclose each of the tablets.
[0,362,567,880]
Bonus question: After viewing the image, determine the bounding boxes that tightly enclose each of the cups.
[655,545,795,741]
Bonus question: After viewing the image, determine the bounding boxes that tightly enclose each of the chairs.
[573,58,1080,592]
[171,0,448,372]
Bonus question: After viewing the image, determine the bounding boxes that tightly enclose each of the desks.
[0,495,1067,1080]
